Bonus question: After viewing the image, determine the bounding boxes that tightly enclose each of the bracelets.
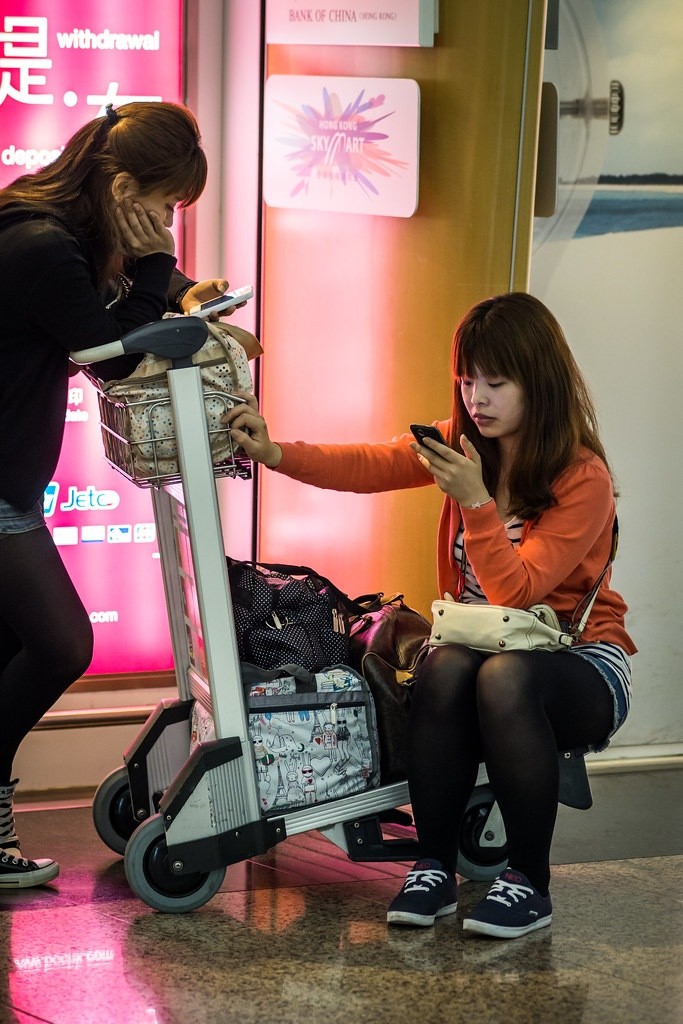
[470,498,493,509]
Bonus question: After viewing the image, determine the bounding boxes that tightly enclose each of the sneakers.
[0,848,59,888]
[386,859,459,927]
[0,778,22,857]
[462,867,552,937]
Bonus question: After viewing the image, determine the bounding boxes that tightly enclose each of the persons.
[222,292,638,938]
[0,102,247,890]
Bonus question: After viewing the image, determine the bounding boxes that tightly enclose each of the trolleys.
[64,313,595,914]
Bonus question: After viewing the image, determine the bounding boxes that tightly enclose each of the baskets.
[96,391,254,492]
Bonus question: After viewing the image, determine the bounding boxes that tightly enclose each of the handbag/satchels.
[239,660,381,816]
[427,591,573,653]
[103,312,264,482]
[349,591,433,786]
[224,555,384,677]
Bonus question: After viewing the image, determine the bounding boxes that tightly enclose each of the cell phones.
[191,285,254,319]
[409,425,448,457]
